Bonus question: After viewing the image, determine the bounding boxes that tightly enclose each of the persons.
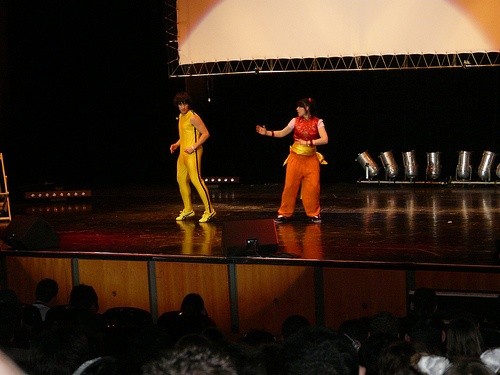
[0,274,500,375]
[169,92,217,222]
[255,97,327,223]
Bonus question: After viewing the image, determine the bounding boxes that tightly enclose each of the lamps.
[355,151,500,184]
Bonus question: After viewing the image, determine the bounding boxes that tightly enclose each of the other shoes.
[312,217,321,222]
[274,217,288,224]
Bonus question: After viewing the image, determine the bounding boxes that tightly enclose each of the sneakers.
[176,209,196,221]
[199,209,216,223]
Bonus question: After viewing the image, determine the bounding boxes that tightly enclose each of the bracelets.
[309,139,313,146]
[271,131,275,137]
[190,145,195,150]
